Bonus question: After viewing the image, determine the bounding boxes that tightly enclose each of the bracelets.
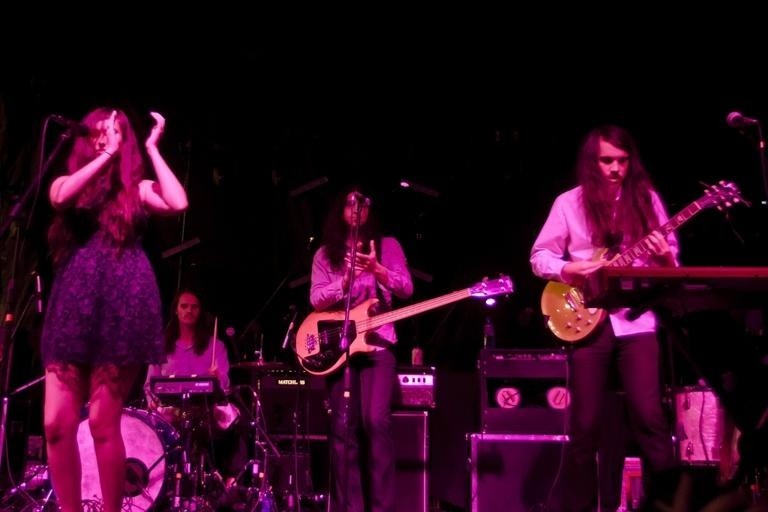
[103,152,113,158]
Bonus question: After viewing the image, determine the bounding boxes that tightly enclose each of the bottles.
[484,318,496,350]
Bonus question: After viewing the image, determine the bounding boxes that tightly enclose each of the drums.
[76,406,190,512]
[673,385,726,469]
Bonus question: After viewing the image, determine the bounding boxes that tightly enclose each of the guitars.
[294,271,514,379]
[542,180,750,345]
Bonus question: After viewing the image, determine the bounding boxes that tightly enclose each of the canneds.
[412,347,424,366]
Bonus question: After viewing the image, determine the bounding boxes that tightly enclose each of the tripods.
[0,372,328,512]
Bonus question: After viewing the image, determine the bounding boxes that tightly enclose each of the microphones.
[727,111,757,127]
[48,113,89,136]
[353,193,371,206]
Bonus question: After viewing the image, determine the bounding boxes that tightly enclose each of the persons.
[526,122,681,509]
[141,287,237,477]
[40,105,187,510]
[313,183,415,510]
